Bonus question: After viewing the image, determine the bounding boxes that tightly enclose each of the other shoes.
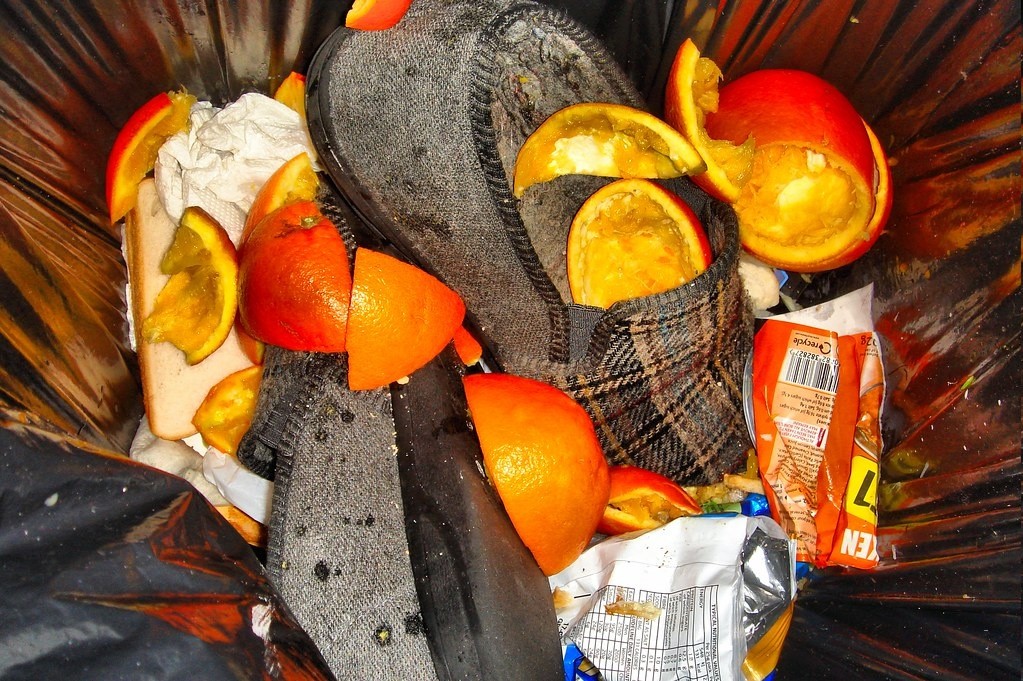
[303,0,754,485]
[235,170,564,681]
